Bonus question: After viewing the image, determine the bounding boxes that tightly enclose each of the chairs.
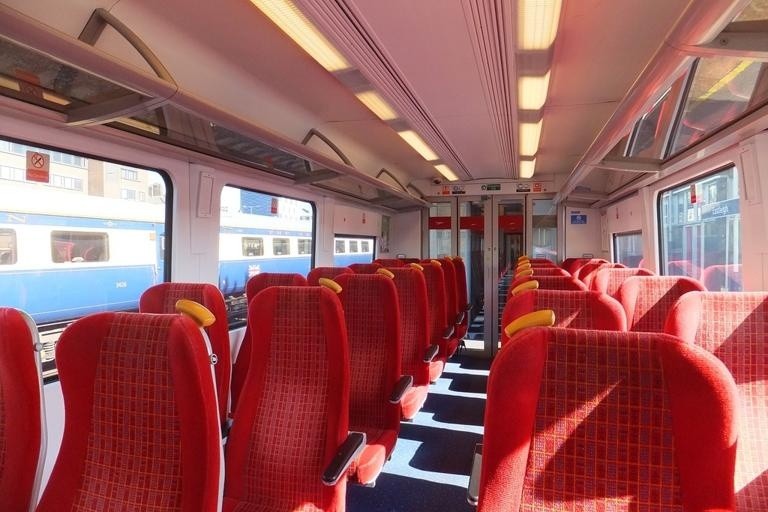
[669,261,703,280]
[505,255,657,300]
[663,291,768,512]
[620,275,711,334]
[0,306,47,512]
[222,278,368,512]
[139,282,232,424]
[700,264,742,292]
[477,309,741,512]
[501,280,627,348]
[35,299,226,512]
